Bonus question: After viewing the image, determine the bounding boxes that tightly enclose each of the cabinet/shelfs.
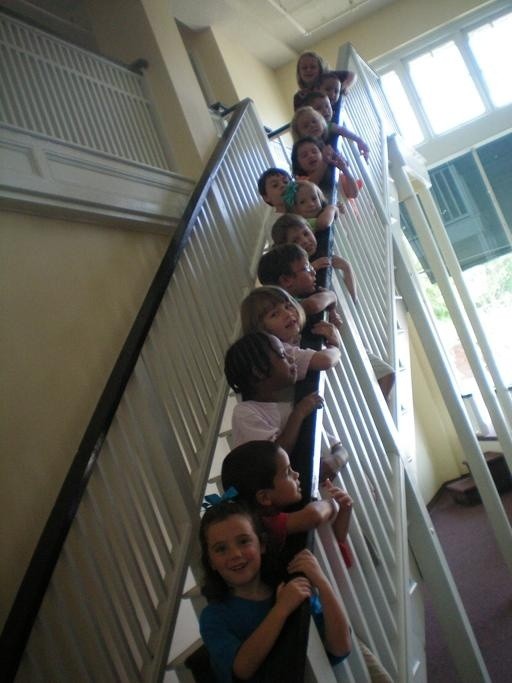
[463,452,512,494]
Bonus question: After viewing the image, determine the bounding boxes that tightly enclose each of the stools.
[447,478,480,505]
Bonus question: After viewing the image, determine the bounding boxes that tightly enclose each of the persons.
[221,440,392,683]
[258,50,371,232]
[224,335,351,486]
[269,213,326,260]
[198,492,352,683]
[239,286,342,384]
[256,243,396,402]
